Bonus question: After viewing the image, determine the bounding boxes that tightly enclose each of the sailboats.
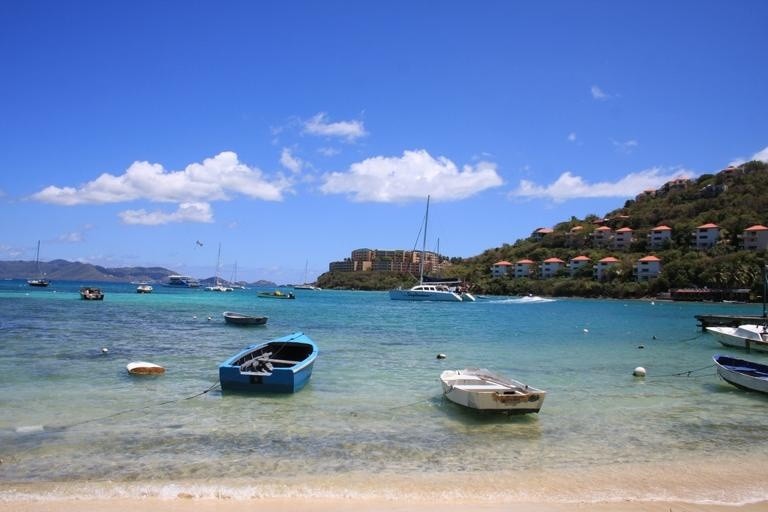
[203,245,235,292]
[229,260,245,288]
[294,257,315,290]
[388,194,477,303]
[26,239,49,287]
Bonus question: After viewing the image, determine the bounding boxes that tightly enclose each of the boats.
[439,365,549,415]
[256,290,296,299]
[136,281,154,294]
[709,352,768,394]
[159,275,203,288]
[222,310,269,326]
[218,328,320,395]
[702,323,768,353]
[79,286,105,301]
